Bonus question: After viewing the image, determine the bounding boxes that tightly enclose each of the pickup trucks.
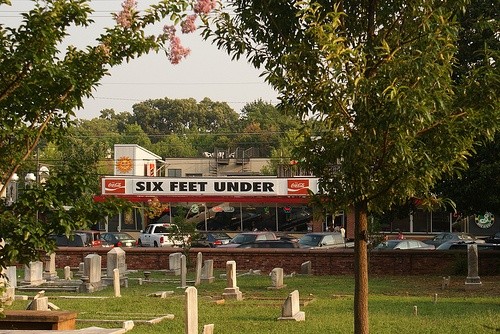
[298,231,355,249]
[215,230,298,248]
[137,223,191,249]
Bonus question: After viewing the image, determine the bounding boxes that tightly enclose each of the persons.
[383,235,387,241]
[397,231,404,240]
[327,223,346,239]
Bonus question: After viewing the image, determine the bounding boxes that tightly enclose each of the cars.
[44,228,108,248]
[189,231,233,249]
[423,231,500,249]
[94,231,138,250]
[373,238,436,249]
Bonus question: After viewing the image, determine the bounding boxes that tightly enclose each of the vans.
[236,240,295,248]
